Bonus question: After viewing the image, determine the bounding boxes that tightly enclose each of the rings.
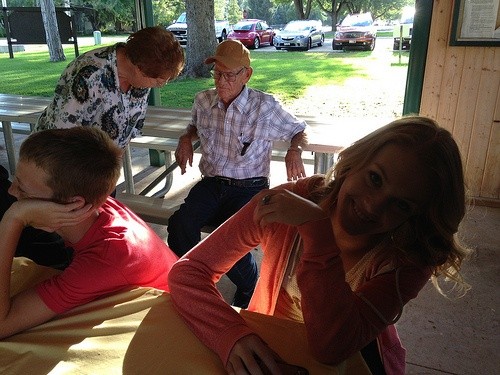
[262,196,269,205]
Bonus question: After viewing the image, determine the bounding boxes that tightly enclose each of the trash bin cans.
[148,149,167,167]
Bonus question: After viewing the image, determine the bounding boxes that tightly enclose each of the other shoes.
[230,288,254,309]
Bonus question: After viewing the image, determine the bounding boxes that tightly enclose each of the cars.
[227,18,276,49]
[273,19,325,51]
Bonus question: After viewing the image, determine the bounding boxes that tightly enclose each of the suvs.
[392,23,413,50]
[332,12,378,51]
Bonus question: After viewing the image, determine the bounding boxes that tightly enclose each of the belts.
[203,175,268,188]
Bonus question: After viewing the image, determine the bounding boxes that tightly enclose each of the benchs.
[0,119,355,233]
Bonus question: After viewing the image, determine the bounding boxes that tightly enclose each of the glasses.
[208,64,246,82]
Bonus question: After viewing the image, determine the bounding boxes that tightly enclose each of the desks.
[0,93,53,123]
[0,256,375,375]
[18,96,348,154]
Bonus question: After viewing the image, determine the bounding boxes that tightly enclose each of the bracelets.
[288,146,302,154]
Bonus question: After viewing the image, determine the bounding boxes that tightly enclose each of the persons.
[0,126,180,338]
[167,116,474,375]
[29,27,185,198]
[167,40,310,309]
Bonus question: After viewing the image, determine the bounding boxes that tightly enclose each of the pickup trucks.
[166,12,230,45]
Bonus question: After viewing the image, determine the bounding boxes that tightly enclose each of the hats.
[205,40,250,69]
